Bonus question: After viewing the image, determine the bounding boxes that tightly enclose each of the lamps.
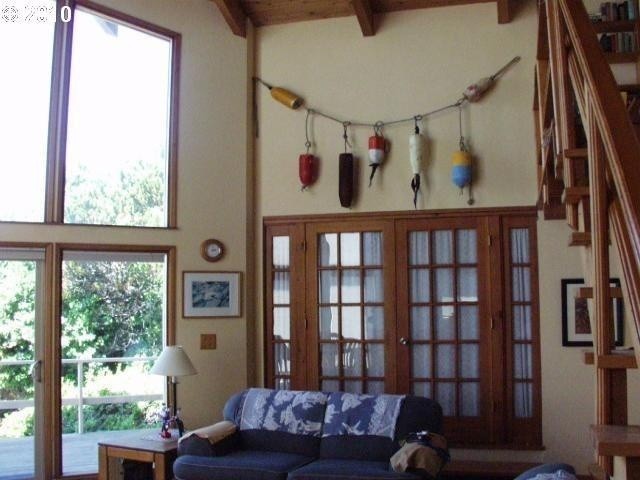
[148,344,199,438]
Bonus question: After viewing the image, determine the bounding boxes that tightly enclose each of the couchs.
[172,388,451,480]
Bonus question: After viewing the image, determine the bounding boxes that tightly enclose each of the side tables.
[98,428,191,480]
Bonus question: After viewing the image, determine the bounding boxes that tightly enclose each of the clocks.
[199,237,225,264]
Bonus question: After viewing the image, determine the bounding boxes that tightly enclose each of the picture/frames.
[559,277,627,347]
[182,270,243,319]
